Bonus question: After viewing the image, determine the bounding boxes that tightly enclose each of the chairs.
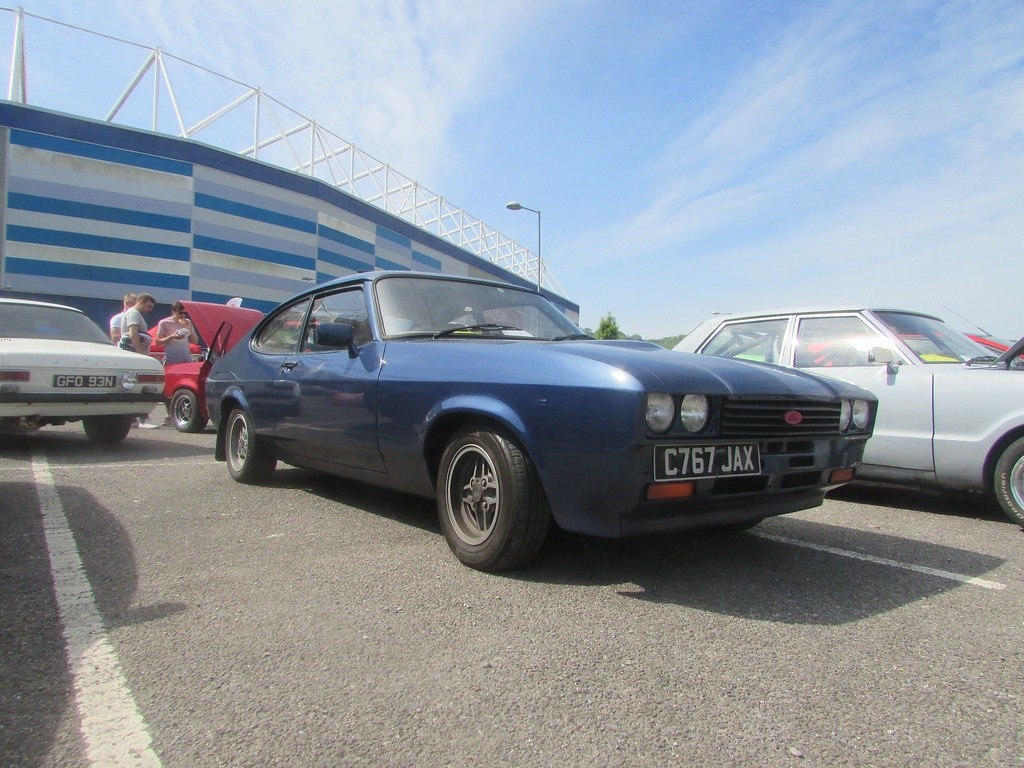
[773,334,782,364]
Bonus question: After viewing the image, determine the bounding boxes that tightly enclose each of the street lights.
[506,201,541,295]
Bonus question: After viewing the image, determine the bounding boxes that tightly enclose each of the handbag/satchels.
[118,330,152,351]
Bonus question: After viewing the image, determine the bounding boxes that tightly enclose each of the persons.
[121,293,159,429]
[110,294,137,346]
[155,300,198,426]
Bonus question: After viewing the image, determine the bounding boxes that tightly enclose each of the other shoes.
[130,419,139,428]
[161,416,174,427]
[139,422,159,429]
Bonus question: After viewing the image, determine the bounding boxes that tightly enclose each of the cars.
[162,298,319,434]
[671,309,1024,525]
[0,297,167,441]
[206,269,879,574]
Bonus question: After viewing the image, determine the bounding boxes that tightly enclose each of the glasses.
[175,309,187,315]
